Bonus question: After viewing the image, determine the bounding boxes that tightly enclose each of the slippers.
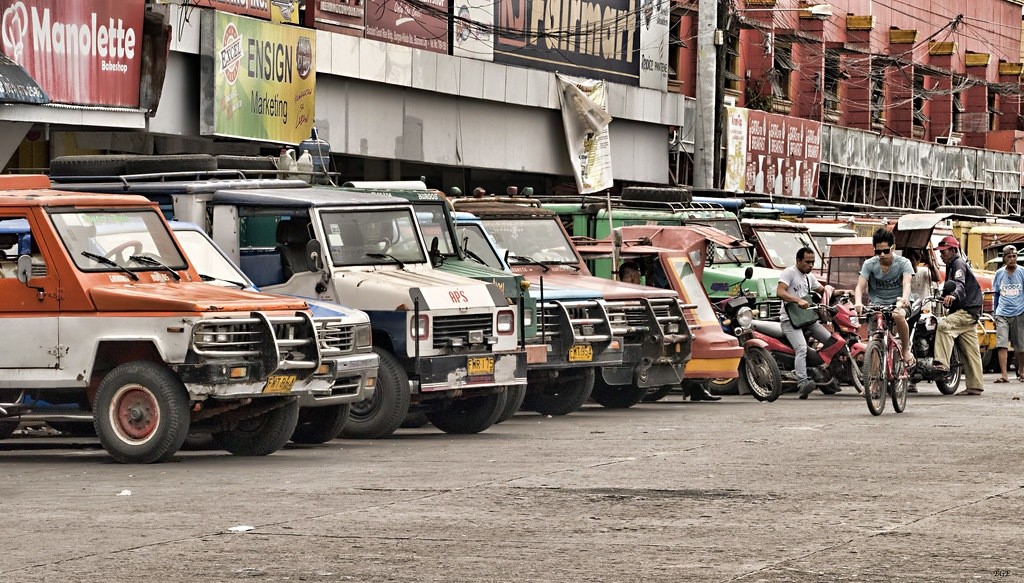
[955,390,980,396]
[903,352,916,368]
[993,378,1009,383]
[932,365,950,372]
[1017,376,1024,382]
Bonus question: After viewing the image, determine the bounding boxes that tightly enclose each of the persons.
[931,236,985,396]
[618,262,721,401]
[992,245,1024,383]
[901,245,941,392]
[776,248,854,400]
[854,227,917,398]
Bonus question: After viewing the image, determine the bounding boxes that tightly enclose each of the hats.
[1002,245,1017,257]
[937,237,959,250]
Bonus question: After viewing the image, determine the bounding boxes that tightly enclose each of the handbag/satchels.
[783,274,819,330]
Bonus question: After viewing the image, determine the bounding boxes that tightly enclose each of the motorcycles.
[704,267,782,406]
[739,281,877,395]
[899,282,965,398]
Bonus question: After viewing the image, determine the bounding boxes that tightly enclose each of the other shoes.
[690,389,722,401]
[829,383,842,392]
[798,378,815,400]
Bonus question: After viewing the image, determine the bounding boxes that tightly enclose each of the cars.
[0,207,378,451]
[35,173,531,440]
[333,181,1023,418]
[0,170,324,460]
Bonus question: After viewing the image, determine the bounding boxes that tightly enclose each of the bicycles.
[846,302,911,415]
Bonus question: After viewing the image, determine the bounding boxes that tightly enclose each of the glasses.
[938,241,951,247]
[874,244,894,255]
